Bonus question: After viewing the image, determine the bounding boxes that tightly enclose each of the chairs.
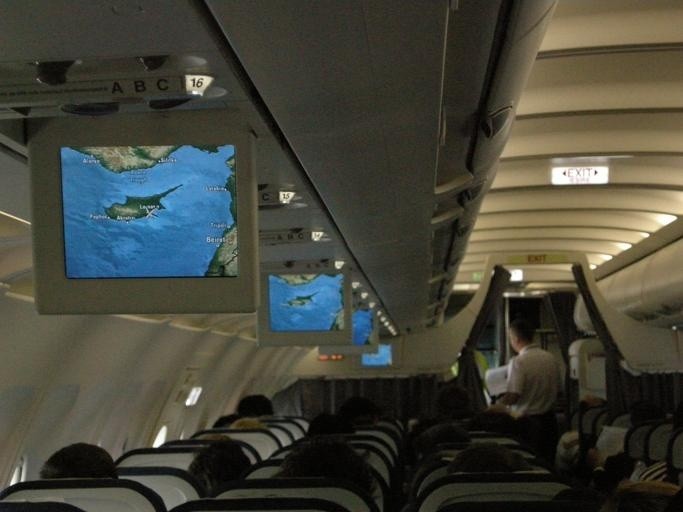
[0,407,682,510]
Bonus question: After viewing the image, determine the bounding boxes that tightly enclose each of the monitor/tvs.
[31,113,260,314]
[318,308,378,354]
[359,343,395,369]
[256,258,350,347]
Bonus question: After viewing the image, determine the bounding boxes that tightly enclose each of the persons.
[495,318,561,463]
[40,443,118,478]
[283,443,373,493]
[630,401,664,425]
[189,440,252,495]
